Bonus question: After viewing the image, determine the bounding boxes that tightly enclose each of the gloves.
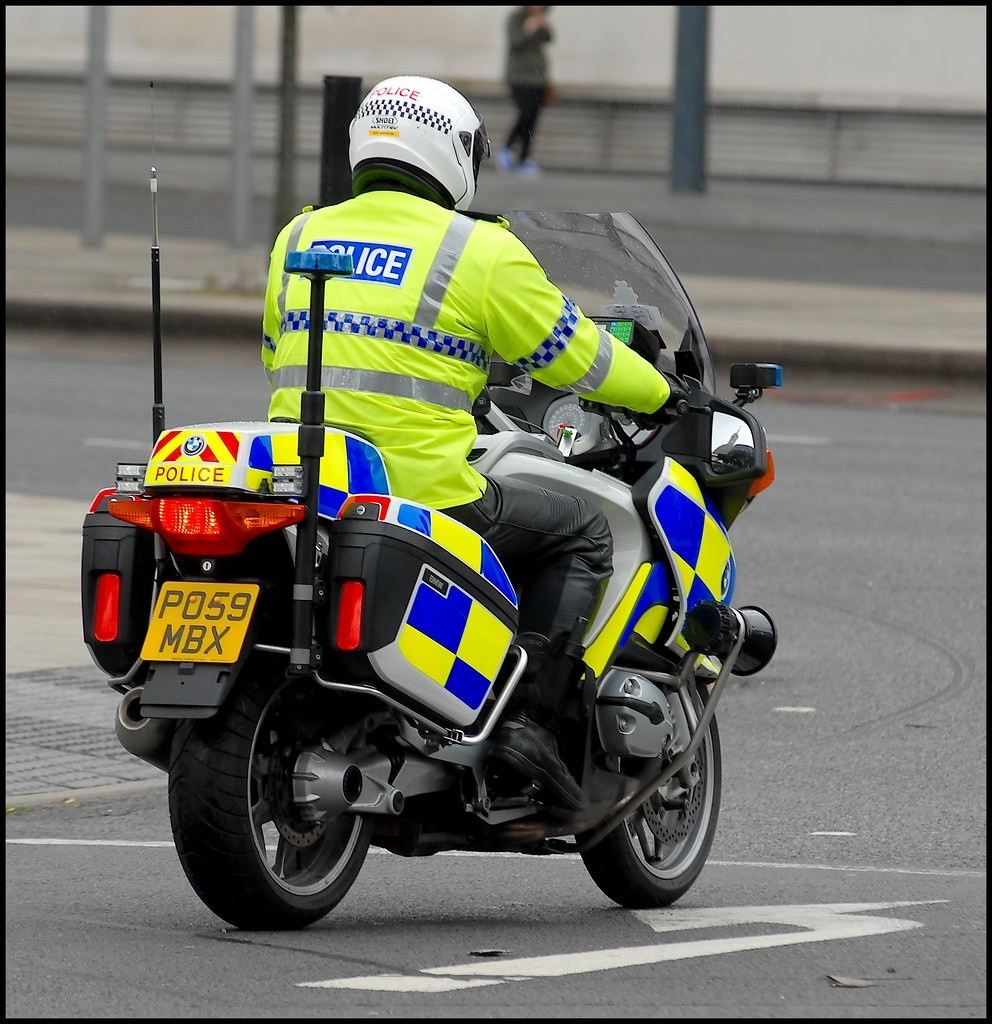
[658,372,691,425]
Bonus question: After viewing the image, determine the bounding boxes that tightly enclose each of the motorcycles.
[75,210,777,926]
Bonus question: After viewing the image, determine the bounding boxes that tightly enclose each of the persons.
[260,75,692,811]
[500,5,553,180]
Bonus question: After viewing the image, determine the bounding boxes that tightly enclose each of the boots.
[486,630,589,812]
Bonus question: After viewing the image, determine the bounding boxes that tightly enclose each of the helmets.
[347,74,490,211]
